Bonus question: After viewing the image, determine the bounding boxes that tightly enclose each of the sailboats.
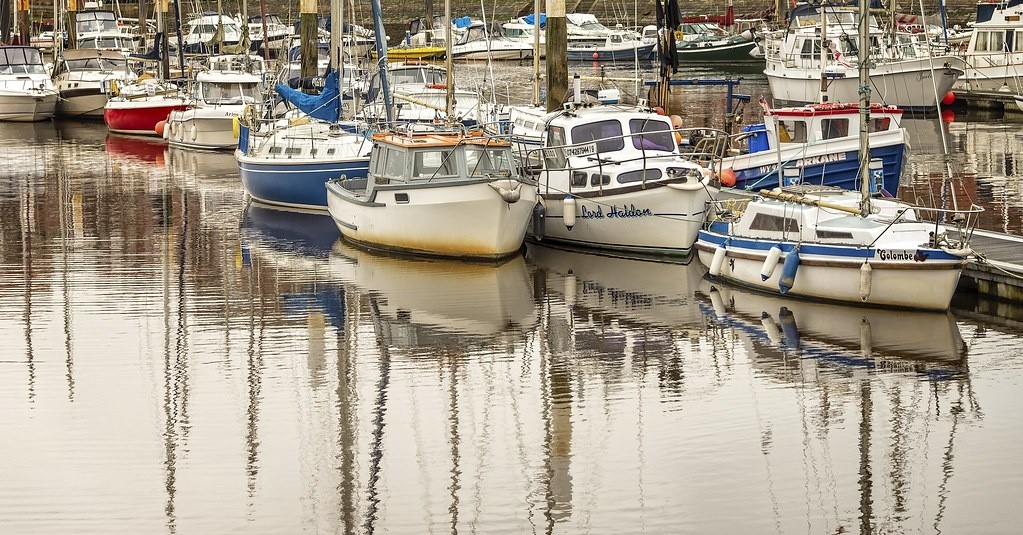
[697,267,974,535]
[0,0,1022,314]
